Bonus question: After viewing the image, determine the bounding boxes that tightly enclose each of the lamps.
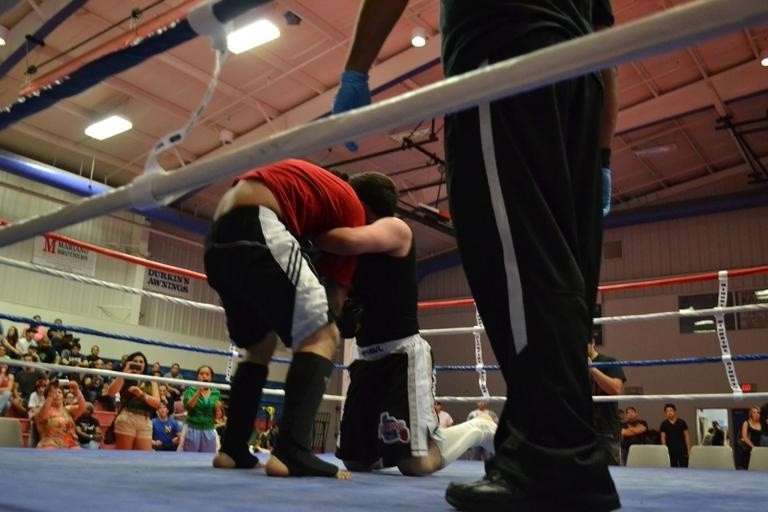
[81,112,135,144]
[410,25,433,48]
[207,18,282,56]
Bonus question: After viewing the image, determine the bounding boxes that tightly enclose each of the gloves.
[332,71,372,151]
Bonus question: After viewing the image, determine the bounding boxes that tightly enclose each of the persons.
[620,406,650,467]
[331,0,620,511]
[710,420,724,444]
[740,404,761,472]
[466,400,498,461]
[583,333,628,470]
[301,172,499,477]
[433,401,454,428]
[0,314,282,454]
[202,157,366,484]
[660,402,692,467]
[758,401,768,447]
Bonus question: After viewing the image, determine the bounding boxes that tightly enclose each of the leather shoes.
[446,460,621,512]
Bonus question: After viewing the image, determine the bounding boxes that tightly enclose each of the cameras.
[54,379,69,387]
[129,364,142,370]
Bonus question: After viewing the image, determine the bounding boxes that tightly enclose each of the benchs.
[1,396,118,450]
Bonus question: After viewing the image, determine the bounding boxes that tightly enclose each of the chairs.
[686,442,738,470]
[746,444,768,471]
[626,443,672,469]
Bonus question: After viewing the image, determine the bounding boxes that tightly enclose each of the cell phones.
[203,379,209,390]
